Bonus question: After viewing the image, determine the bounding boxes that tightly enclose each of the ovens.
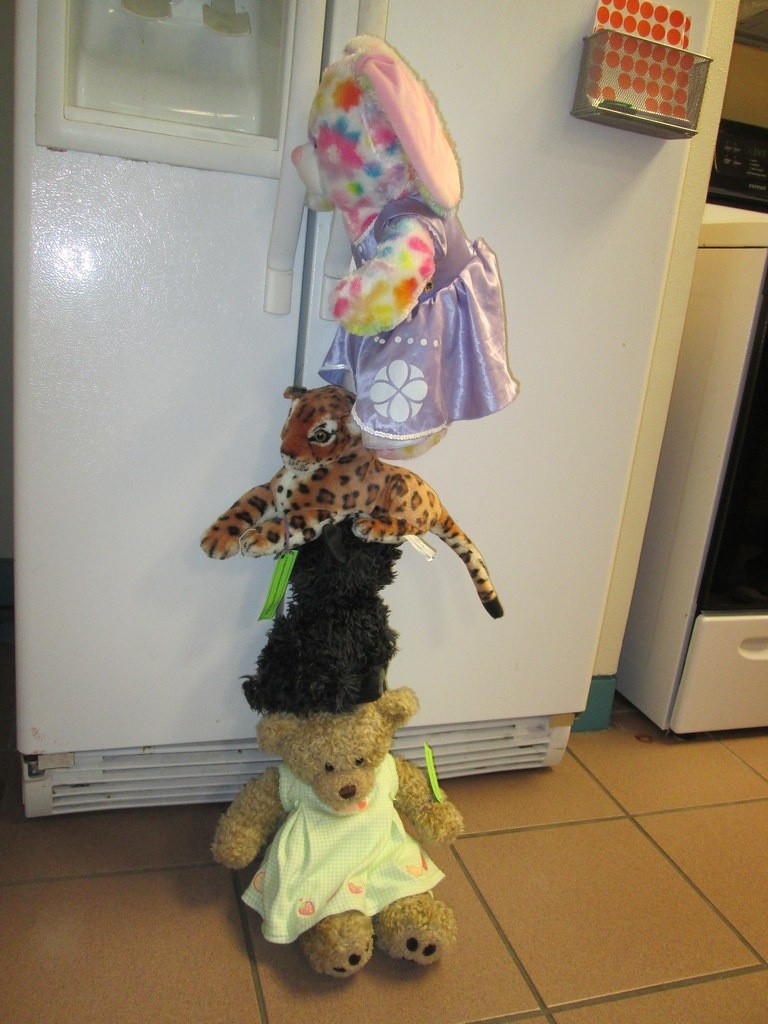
[615,216,767,737]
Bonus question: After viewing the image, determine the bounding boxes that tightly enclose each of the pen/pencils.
[598,99,691,128]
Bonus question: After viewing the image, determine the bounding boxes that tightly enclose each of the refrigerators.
[3,0,739,822]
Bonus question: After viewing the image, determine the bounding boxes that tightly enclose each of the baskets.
[568,28,715,140]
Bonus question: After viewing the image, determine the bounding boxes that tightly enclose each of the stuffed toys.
[240,516,398,714]
[210,684,466,976]
[193,386,503,618]
[290,34,519,460]
